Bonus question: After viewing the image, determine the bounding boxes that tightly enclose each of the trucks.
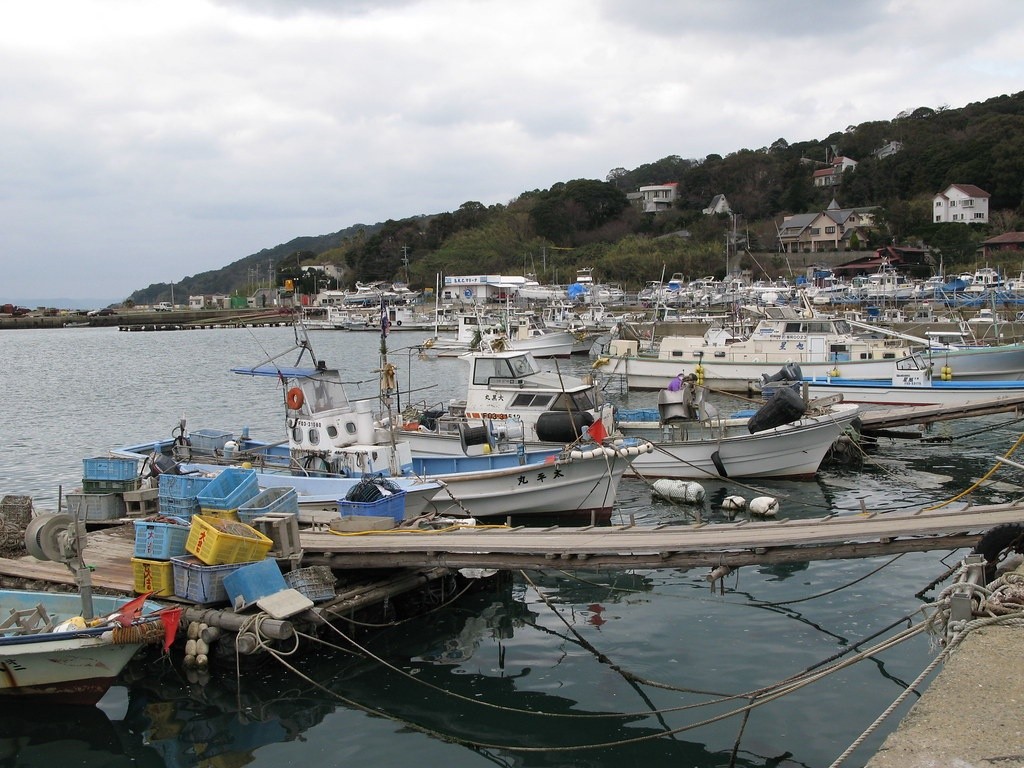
[153,302,173,312]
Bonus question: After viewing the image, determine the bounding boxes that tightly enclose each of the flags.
[587,418,608,446]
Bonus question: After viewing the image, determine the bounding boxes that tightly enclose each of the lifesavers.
[286,385,305,411]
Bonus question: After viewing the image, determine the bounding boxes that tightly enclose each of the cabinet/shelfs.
[0,604,54,637]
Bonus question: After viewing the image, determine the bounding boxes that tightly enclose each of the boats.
[0,485,189,690]
[104,209,1024,518]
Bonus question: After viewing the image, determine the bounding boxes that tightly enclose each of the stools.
[275,549,304,572]
[123,487,160,518]
[251,512,301,557]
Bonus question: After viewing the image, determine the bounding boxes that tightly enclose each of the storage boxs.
[731,381,801,418]
[403,420,418,431]
[830,353,850,361]
[130,468,335,620]
[62,457,143,521]
[613,408,660,422]
[336,490,408,522]
[190,428,233,455]
[0,495,33,524]
